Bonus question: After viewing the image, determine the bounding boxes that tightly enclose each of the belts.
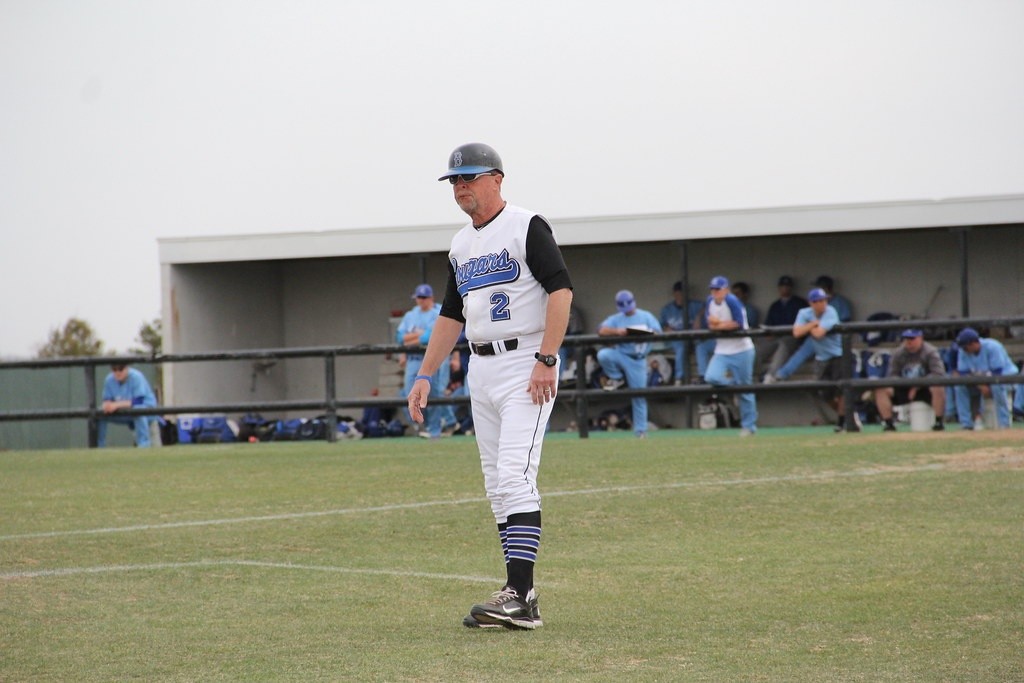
[472,338,518,356]
[629,354,645,360]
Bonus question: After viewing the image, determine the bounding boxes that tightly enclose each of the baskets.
[834,425,843,433]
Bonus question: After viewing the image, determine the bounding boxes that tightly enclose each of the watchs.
[535,351,558,368]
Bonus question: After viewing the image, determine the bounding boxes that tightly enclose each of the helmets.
[438,143,504,181]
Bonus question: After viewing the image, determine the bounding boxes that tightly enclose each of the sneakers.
[463,599,543,628]
[470,585,535,630]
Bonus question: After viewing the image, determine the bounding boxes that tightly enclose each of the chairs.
[155,310,1022,448]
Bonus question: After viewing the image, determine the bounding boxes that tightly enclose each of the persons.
[405,141,575,631]
[395,273,1024,438]
[96,361,158,447]
[597,288,664,440]
[702,277,759,437]
[876,326,947,431]
[792,290,842,436]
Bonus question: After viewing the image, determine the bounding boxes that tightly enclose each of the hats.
[615,291,635,311]
[778,276,793,285]
[416,285,432,297]
[709,276,728,288]
[810,276,833,288]
[955,327,978,343]
[901,329,923,337]
[673,281,683,289]
[807,289,829,302]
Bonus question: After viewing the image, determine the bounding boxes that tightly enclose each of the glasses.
[417,296,428,299]
[449,173,498,184]
[112,366,126,372]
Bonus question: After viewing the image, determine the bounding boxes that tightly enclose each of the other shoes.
[418,423,473,438]
[883,425,896,431]
[932,422,944,430]
[762,374,776,384]
[739,428,752,438]
[604,378,624,390]
[675,376,707,386]
[635,431,647,439]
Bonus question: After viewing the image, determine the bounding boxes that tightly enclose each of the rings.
[544,390,551,392]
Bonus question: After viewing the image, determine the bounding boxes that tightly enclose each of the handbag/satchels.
[160,406,404,446]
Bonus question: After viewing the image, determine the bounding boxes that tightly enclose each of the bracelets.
[415,375,435,385]
